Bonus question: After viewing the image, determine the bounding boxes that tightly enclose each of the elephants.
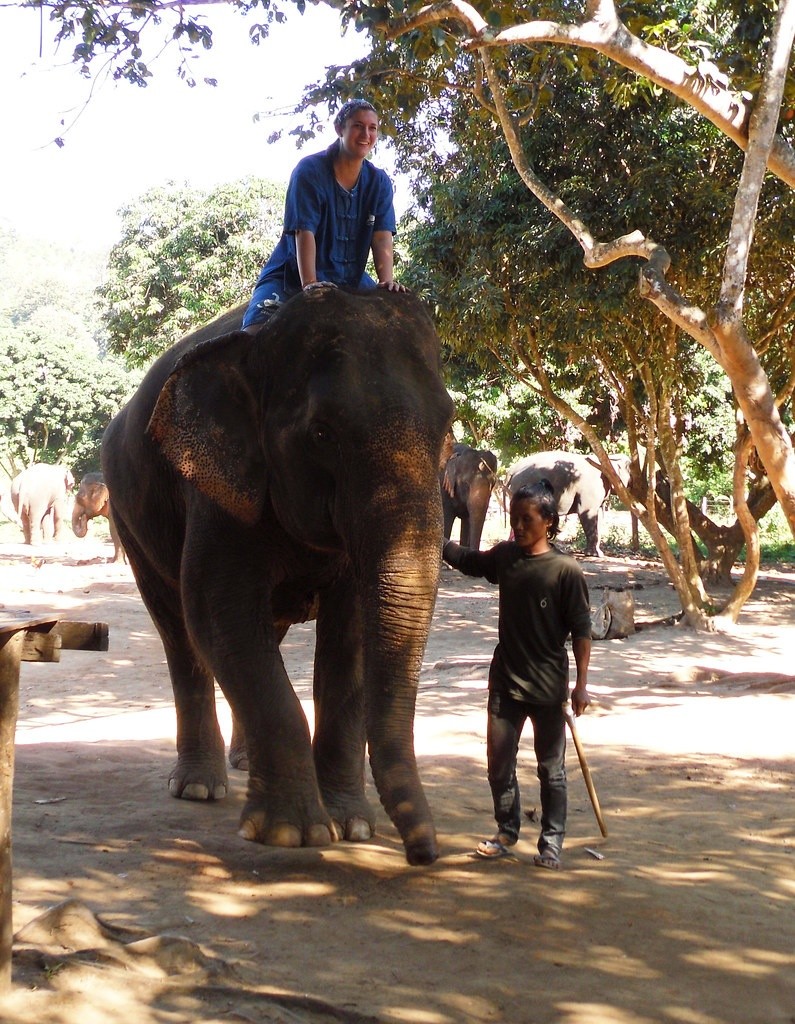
[439,442,634,580]
[10,462,130,565]
[101,280,457,868]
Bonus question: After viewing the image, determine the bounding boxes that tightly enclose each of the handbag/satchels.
[591,602,611,640]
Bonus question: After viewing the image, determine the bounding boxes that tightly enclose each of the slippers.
[477,840,513,858]
[534,855,560,869]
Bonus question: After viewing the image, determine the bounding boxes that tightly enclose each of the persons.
[443,478,592,871]
[242,100,412,337]
